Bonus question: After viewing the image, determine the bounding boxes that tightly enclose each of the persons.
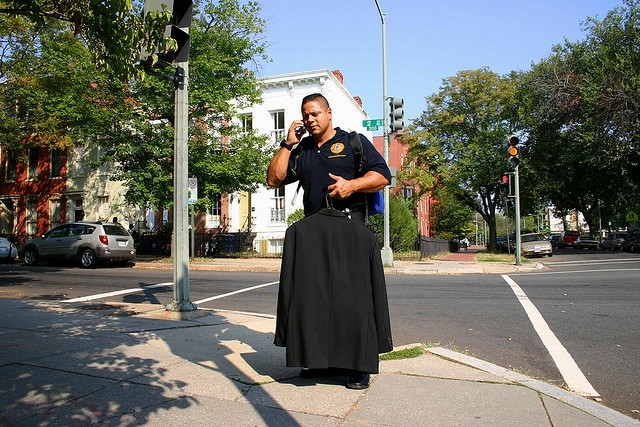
[113,217,118,223]
[267,91,392,391]
[461,236,468,249]
[129,224,140,247]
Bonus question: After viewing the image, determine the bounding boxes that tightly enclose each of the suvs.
[18,221,137,268]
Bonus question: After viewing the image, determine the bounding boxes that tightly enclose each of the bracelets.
[280,140,292,151]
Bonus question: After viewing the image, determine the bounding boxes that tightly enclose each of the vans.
[560,230,582,248]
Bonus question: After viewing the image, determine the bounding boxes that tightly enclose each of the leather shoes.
[346,370,369,388]
[299,366,333,376]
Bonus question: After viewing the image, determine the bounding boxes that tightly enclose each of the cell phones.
[302,126,307,134]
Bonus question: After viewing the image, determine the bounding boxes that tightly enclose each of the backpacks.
[348,130,385,216]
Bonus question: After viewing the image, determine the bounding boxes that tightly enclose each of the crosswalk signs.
[626,210,638,222]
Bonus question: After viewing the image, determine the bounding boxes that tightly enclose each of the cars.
[621,232,640,253]
[520,233,553,258]
[496,237,506,245]
[572,236,599,251]
[550,235,564,249]
[0,237,17,261]
[598,233,628,253]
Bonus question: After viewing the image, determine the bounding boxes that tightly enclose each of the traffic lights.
[387,94,404,133]
[500,173,510,184]
[507,135,520,168]
[136,0,192,85]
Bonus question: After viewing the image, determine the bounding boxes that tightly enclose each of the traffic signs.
[362,118,382,130]
